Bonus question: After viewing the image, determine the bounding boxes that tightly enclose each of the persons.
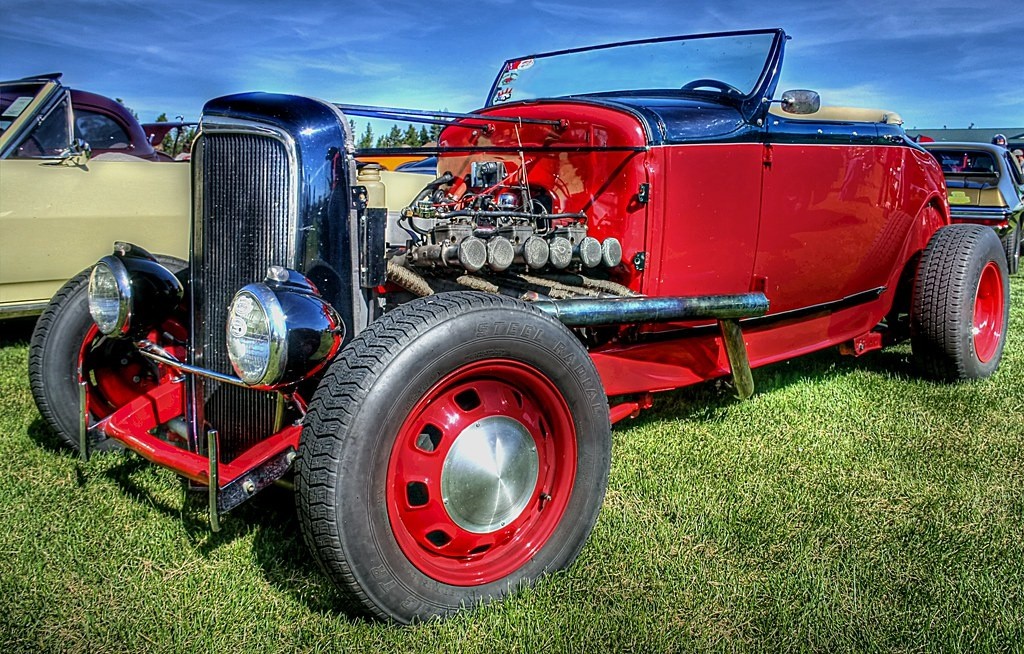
[974,134,1024,175]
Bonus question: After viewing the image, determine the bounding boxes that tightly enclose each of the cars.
[30,29,1023,627]
[0,72,447,325]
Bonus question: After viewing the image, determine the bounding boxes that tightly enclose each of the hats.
[1012,149,1023,155]
[992,134,1008,146]
